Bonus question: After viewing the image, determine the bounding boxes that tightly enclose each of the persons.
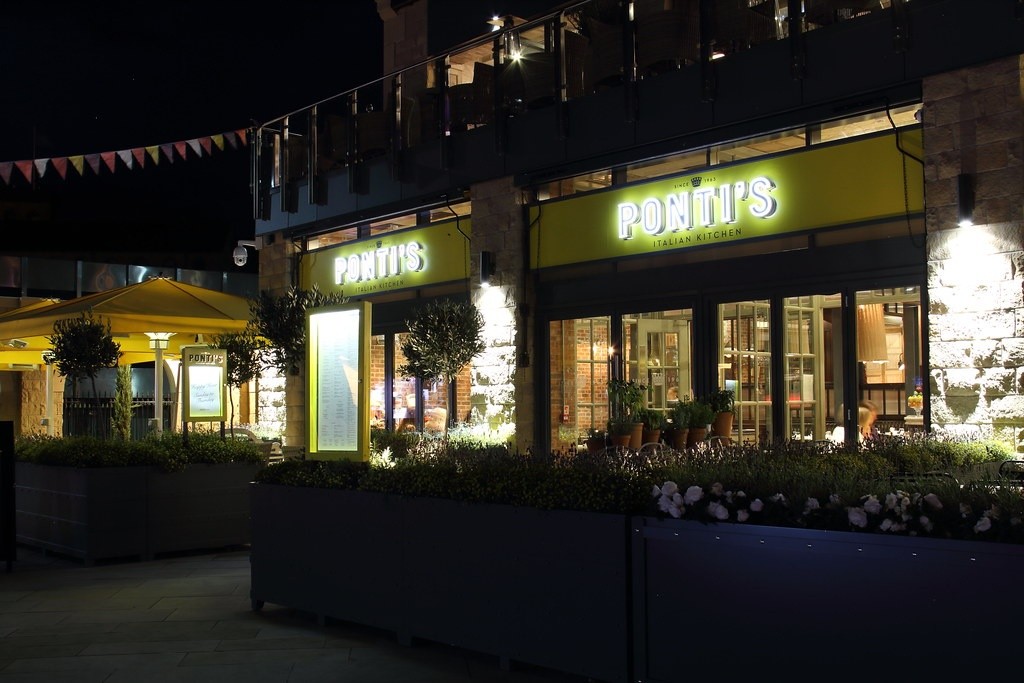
[859,401,880,440]
[398,406,417,433]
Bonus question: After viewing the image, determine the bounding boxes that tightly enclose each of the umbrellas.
[0,274,270,438]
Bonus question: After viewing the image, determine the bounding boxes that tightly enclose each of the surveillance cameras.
[233,246,247,266]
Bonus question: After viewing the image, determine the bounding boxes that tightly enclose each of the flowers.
[641,436,1023,543]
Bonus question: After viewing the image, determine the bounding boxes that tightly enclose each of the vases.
[632,516,1023,683]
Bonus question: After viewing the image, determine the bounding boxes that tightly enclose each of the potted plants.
[12,429,268,568]
[249,443,632,683]
[586,378,736,452]
